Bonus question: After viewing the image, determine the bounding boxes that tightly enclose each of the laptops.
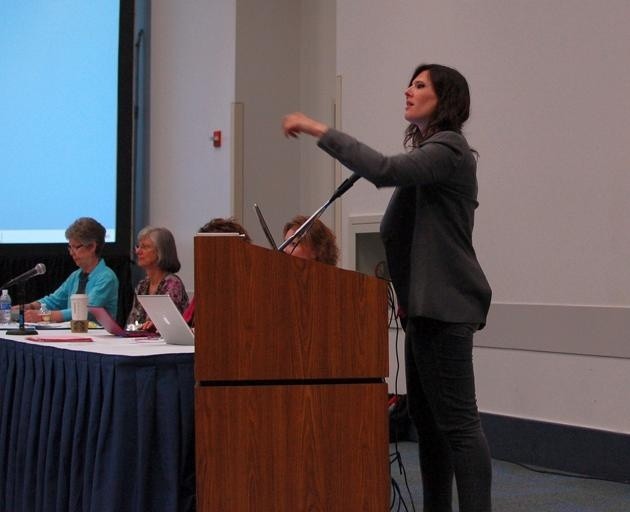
[136,294,195,346]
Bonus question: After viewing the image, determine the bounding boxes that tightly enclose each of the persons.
[156,217,253,338]
[124,226,190,336]
[283,215,340,266]
[281,63,493,512]
[9,217,120,328]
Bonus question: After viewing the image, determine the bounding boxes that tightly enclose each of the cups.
[70,294,89,333]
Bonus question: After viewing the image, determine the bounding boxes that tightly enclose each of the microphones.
[329,172,364,204]
[8,263,46,286]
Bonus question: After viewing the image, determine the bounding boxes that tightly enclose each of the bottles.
[0,289,11,326]
[38,303,50,315]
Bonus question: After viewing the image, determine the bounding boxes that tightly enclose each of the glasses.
[67,245,82,252]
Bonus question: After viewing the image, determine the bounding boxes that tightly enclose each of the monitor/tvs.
[252,202,280,251]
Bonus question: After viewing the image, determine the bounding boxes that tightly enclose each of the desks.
[0,322,196,512]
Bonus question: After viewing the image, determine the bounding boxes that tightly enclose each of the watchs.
[42,311,52,322]
